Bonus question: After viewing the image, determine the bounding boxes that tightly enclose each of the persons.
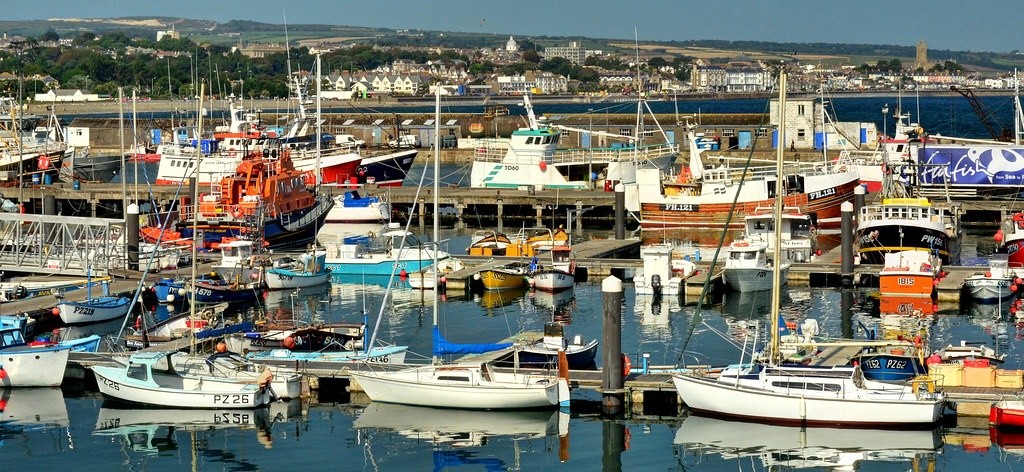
[797,139,799,152]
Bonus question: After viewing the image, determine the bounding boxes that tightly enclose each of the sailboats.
[90,76,272,409]
[90,399,266,472]
[343,83,561,408]
[352,400,560,472]
[670,62,959,431]
[671,414,945,472]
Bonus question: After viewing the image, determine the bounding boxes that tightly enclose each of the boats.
[996,401,1024,428]
[0,10,1023,335]
[148,40,421,226]
[0,346,73,388]
[0,388,74,434]
[995,428,1024,455]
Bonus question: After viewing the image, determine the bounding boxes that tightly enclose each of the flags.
[779,312,791,336]
[900,146,915,163]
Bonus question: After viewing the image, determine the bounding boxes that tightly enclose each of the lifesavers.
[912,458,936,472]
[621,354,631,377]
[228,148,237,156]
[733,242,750,247]
[624,427,632,450]
[230,204,244,218]
[912,376,935,394]
[809,226,817,236]
